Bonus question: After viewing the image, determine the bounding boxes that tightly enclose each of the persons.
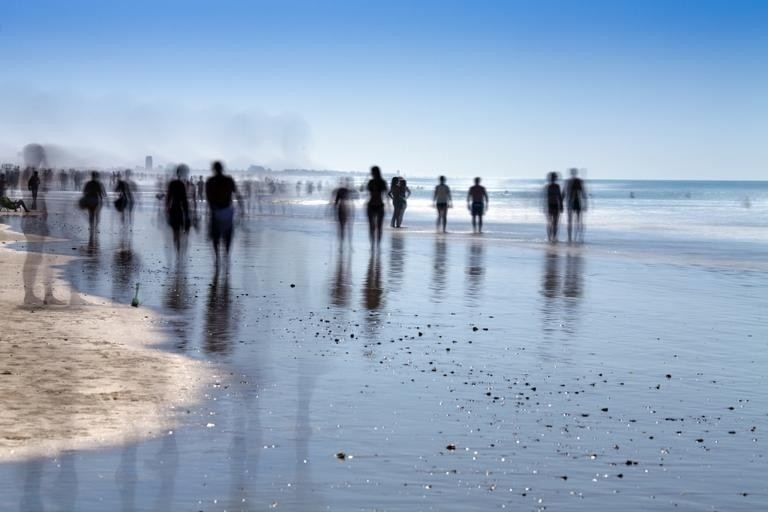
[0,142,587,308]
